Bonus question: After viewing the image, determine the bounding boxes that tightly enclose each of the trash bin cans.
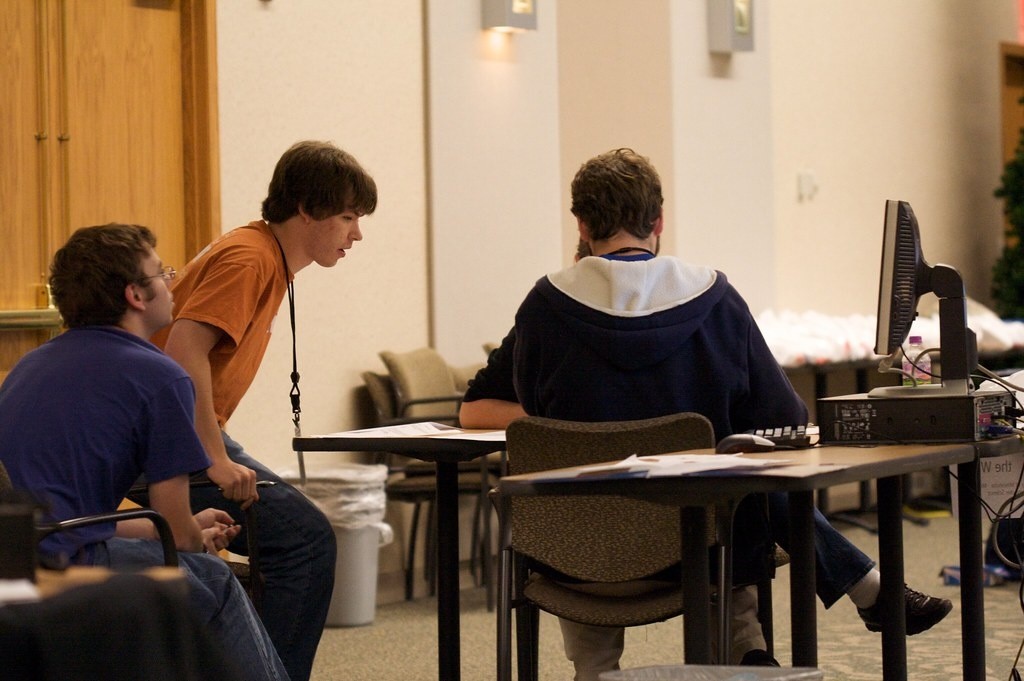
[274,464,389,628]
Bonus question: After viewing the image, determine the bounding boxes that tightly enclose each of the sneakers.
[857,581,953,637]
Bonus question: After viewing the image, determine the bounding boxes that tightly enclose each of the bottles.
[902,336,931,385]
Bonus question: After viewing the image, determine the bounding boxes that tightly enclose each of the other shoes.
[739,650,778,667]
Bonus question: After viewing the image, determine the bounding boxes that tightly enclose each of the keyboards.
[742,425,820,447]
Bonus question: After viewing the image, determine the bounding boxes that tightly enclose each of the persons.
[457,232,952,666]
[146,140,379,681]
[0,222,293,681]
[513,147,810,681]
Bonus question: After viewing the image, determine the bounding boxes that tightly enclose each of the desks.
[295,418,512,681]
[501,433,1023,681]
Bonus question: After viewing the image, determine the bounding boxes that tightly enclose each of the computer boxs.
[815,390,1019,445]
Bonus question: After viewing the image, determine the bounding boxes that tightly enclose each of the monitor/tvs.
[866,200,979,398]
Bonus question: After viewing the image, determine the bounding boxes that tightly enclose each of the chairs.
[0,475,277,681]
[362,341,793,681]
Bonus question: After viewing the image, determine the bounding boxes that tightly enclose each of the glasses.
[135,266,177,283]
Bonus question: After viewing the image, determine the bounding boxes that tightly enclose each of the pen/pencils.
[217,481,279,491]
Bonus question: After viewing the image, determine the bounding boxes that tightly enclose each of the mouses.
[714,434,776,454]
